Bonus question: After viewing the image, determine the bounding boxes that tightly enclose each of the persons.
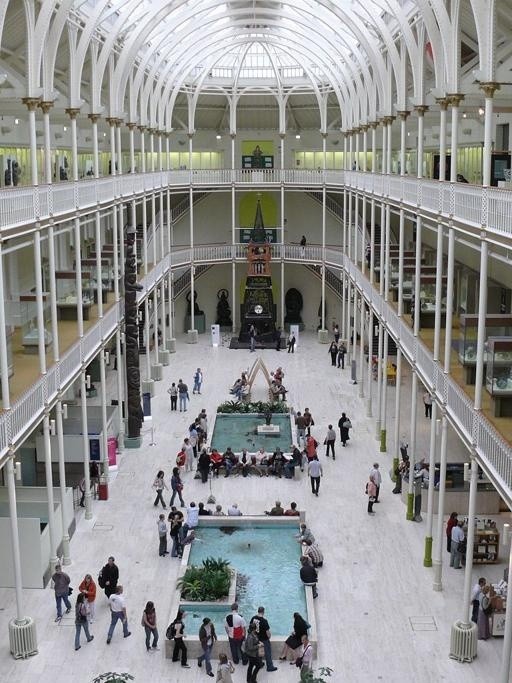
[423,392,432,419]
[394,455,429,492]
[367,464,381,513]
[338,413,351,447]
[308,455,322,496]
[300,235,306,246]
[80,461,98,506]
[469,577,494,640]
[327,325,361,369]
[248,325,296,353]
[446,512,465,569]
[231,366,287,403]
[152,467,241,557]
[168,367,202,412]
[52,556,131,651]
[144,601,159,650]
[296,523,323,598]
[176,407,319,484]
[265,501,300,516]
[172,602,314,683]
[325,424,336,460]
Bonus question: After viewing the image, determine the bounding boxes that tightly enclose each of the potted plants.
[177,554,237,611]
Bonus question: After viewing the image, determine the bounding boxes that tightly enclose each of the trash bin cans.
[98,481,109,500]
[143,392,151,417]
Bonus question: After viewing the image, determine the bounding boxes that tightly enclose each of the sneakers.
[171,655,295,682]
[53,607,130,650]
[147,646,159,653]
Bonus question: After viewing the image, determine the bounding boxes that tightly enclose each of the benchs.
[206,457,297,477]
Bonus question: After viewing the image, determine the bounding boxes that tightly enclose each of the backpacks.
[165,620,181,639]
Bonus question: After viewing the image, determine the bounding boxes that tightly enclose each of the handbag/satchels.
[483,606,492,615]
[296,657,302,667]
[456,543,465,552]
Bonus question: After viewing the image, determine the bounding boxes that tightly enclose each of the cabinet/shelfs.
[363,241,512,418]
[456,514,501,564]
[9,226,142,353]
